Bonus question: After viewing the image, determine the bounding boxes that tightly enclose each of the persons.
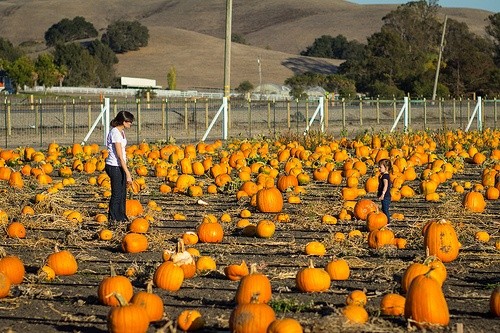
[105,110,135,229]
[377,159,393,225]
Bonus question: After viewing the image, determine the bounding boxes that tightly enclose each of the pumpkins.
[0,130,500,333]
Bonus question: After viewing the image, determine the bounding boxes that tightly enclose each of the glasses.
[125,120,132,124]
